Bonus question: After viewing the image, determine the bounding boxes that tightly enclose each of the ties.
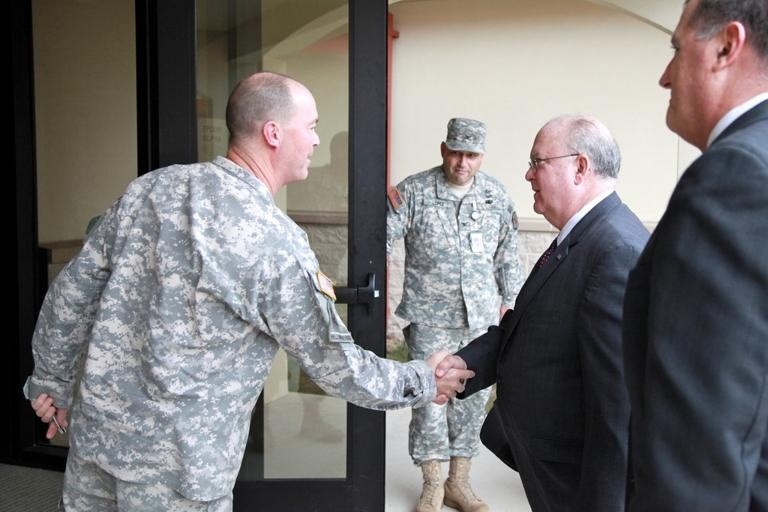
[539,237,560,275]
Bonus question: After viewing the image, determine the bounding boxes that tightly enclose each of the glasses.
[529,153,584,169]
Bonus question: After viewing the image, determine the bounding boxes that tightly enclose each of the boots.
[414,456,488,511]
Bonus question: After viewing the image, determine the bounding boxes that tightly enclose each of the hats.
[444,116,488,154]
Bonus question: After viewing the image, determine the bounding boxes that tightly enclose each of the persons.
[381,113,528,511]
[617,1,768,510]
[429,113,652,511]
[20,71,477,512]
[284,127,349,405]
